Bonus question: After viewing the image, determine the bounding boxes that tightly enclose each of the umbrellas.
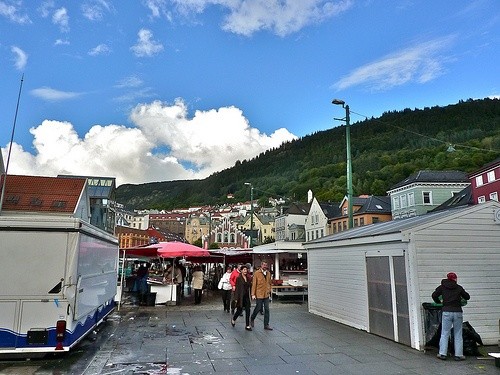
[134,241,211,283]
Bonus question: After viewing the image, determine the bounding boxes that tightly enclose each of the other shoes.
[231,318,235,327]
[455,356,465,361]
[245,325,253,331]
[264,325,273,330]
[436,353,447,360]
[251,320,255,327]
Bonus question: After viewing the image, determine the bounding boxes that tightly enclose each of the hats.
[447,272,457,280]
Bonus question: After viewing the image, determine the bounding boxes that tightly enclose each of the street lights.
[332,99,353,229]
[244,183,253,248]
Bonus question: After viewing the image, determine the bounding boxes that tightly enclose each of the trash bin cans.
[423,299,444,349]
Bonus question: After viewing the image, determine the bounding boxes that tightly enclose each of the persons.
[251,258,273,330]
[432,272,470,360]
[231,266,252,330]
[125,260,271,316]
